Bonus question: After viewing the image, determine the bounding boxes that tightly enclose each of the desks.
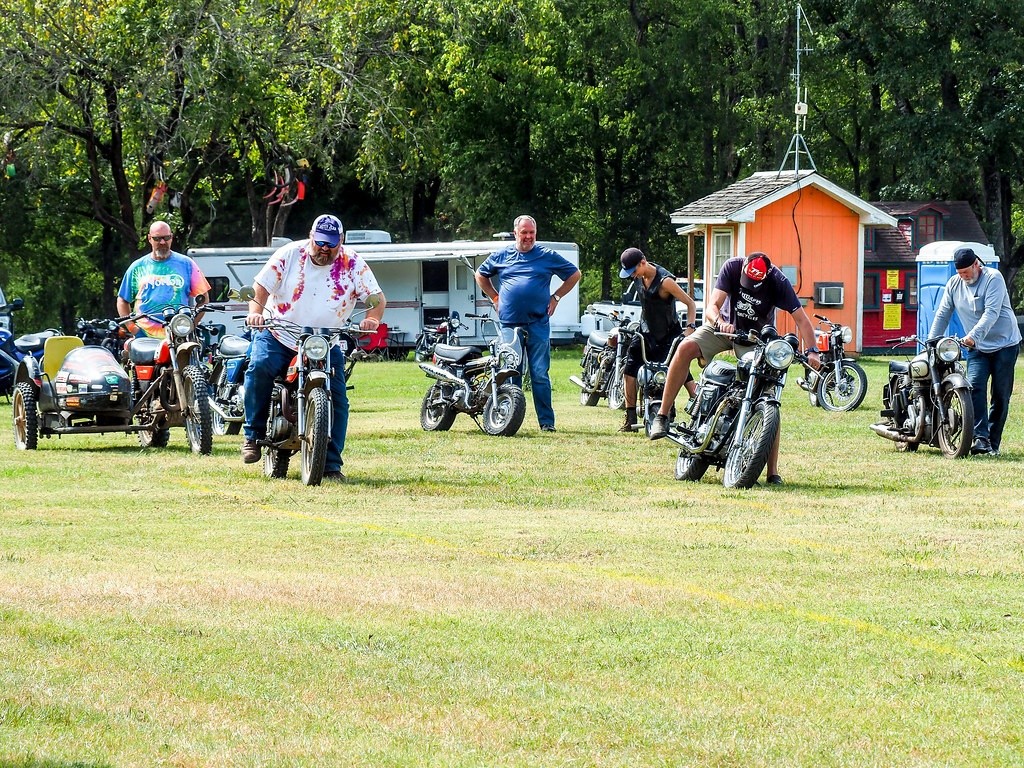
[387,330,409,361]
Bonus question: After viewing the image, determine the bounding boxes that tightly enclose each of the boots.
[617,408,639,433]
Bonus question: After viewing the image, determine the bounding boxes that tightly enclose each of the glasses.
[149,235,171,242]
[314,241,337,248]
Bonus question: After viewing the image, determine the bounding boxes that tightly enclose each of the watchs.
[687,323,696,330]
[805,346,819,353]
[552,294,561,302]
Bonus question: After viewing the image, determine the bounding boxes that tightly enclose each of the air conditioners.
[819,287,844,304]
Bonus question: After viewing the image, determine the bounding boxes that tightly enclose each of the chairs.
[359,324,388,362]
[44,336,85,382]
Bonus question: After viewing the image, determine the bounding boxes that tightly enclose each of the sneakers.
[650,414,669,440]
[766,475,782,483]
[969,439,992,455]
[241,439,261,463]
[986,450,1000,456]
[323,472,347,481]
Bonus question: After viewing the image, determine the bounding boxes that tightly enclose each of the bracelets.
[492,295,499,303]
[366,317,379,324]
[128,324,135,331]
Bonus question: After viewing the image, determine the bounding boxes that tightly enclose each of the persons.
[474,215,581,433]
[928,248,1022,456]
[617,248,697,434]
[242,215,387,483]
[650,251,821,489]
[116,222,211,342]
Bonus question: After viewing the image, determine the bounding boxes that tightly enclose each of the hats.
[311,213,343,245]
[953,248,976,268]
[740,252,771,291]
[618,247,643,277]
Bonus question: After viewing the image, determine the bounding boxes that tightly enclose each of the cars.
[674,277,703,329]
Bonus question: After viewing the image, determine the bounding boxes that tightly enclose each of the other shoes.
[541,424,556,432]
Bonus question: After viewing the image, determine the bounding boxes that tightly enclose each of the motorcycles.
[12,294,225,456]
[617,328,676,437]
[97,313,140,362]
[413,311,469,362]
[70,316,110,346]
[664,326,830,489]
[207,315,246,435]
[417,313,528,438]
[869,334,975,459]
[796,314,868,412]
[0,288,66,405]
[231,286,379,487]
[570,312,640,408]
